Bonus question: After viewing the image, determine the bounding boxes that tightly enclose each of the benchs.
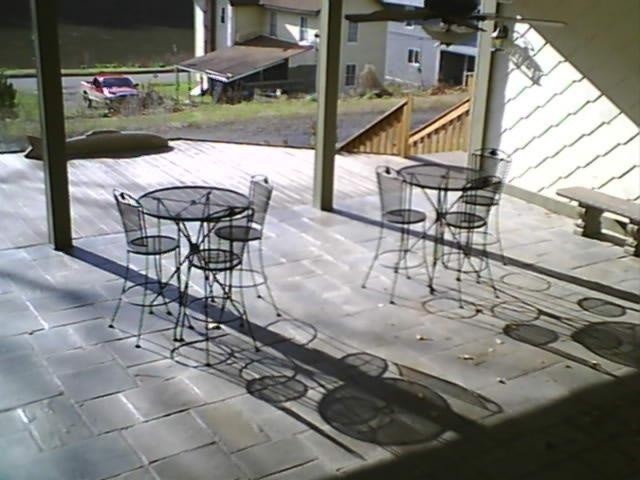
[557,186,639,255]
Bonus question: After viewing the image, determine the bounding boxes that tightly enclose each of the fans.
[344,0,568,32]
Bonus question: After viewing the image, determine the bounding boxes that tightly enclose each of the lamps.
[489,25,508,50]
[421,23,473,45]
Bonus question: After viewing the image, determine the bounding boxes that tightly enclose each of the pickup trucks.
[78,70,141,110]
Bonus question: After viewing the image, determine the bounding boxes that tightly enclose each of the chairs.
[108,174,282,368]
[360,148,509,307]
